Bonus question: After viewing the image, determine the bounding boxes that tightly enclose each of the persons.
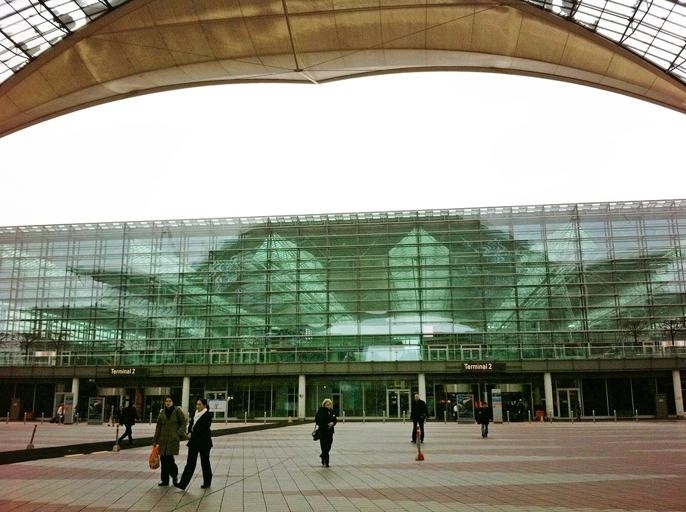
[118,401,140,444]
[315,399,337,467]
[476,403,490,438]
[152,396,187,486]
[411,394,428,444]
[175,397,214,490]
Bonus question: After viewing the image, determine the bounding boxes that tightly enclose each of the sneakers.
[174,482,187,491]
[201,484,211,489]
[157,481,169,487]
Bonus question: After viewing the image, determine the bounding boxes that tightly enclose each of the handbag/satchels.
[149,445,161,468]
[312,424,320,441]
